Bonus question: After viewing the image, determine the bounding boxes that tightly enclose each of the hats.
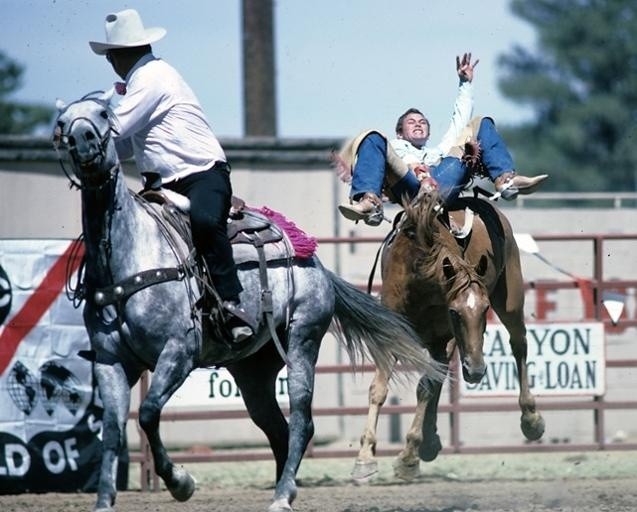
[88,9,167,55]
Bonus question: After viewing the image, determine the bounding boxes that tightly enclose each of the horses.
[49,85,452,512]
[349,195,546,484]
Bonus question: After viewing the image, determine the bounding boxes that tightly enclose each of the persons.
[338,52,549,226]
[77,9,253,362]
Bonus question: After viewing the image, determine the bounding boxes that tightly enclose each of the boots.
[495,172,549,201]
[338,192,384,226]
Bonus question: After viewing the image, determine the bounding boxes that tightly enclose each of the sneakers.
[78,351,95,360]
[225,302,254,343]
